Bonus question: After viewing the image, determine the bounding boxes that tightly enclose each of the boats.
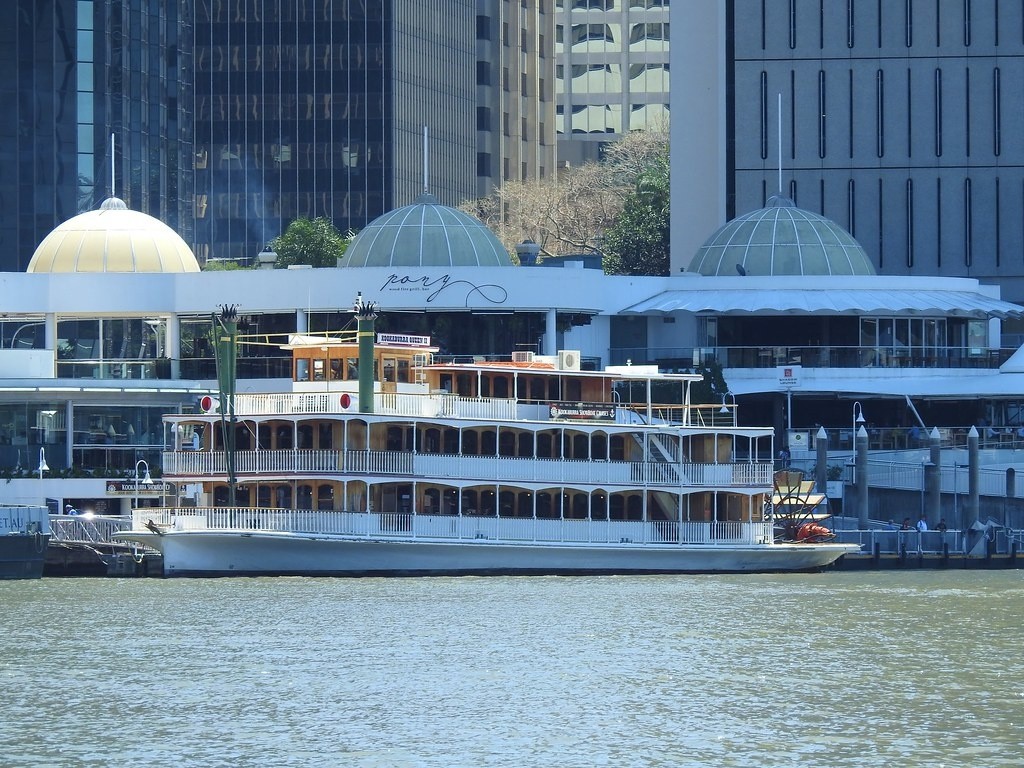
[113,298,859,573]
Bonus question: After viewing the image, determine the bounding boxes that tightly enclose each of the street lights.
[135,461,153,509]
[852,402,869,489]
[40,447,49,479]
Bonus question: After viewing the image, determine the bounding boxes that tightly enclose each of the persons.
[66,504,77,516]
[891,416,1024,449]
[882,514,947,554]
